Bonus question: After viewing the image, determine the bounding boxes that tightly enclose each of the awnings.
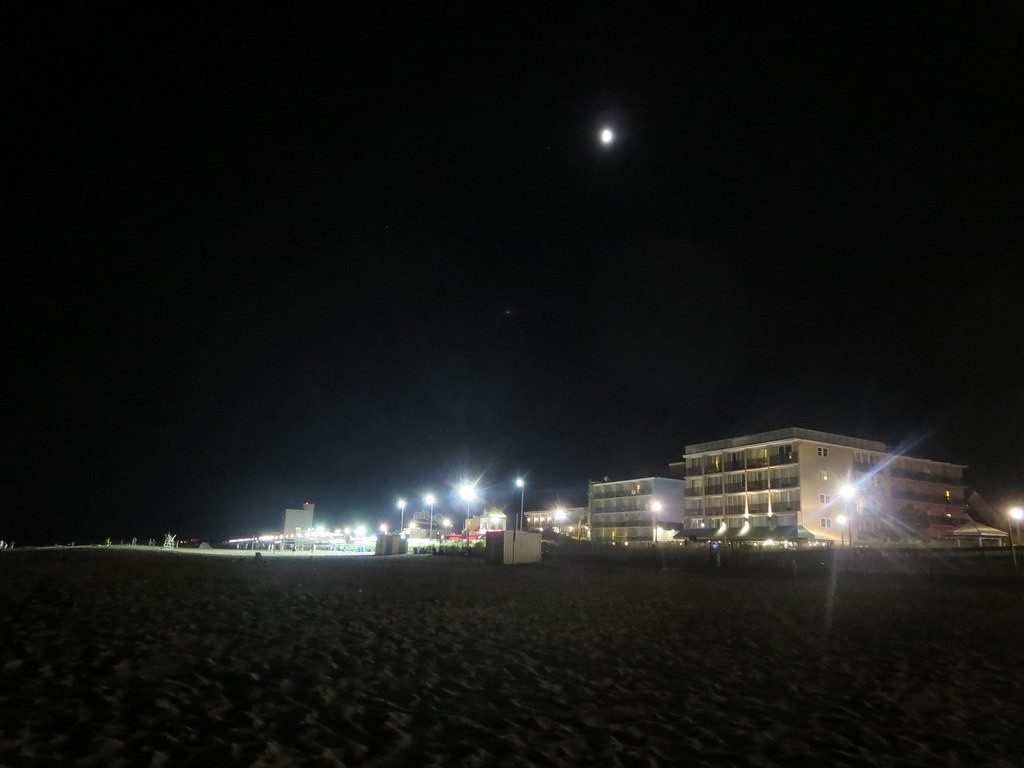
[673,526,814,542]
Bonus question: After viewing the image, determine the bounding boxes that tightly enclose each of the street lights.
[1006,507,1024,574]
[420,491,438,537]
[449,477,485,548]
[650,501,662,543]
[513,477,528,530]
[553,509,569,535]
[837,483,856,547]
[395,497,408,529]
